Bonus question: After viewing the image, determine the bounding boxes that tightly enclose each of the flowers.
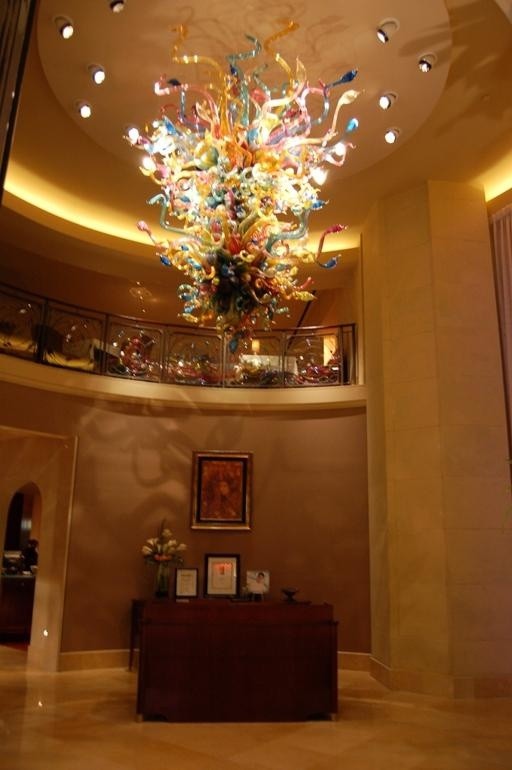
[141,529,187,569]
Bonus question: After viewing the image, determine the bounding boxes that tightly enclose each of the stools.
[128,600,146,671]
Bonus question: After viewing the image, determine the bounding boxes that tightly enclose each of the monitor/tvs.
[3,550,24,568]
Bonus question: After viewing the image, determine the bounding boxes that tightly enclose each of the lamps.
[377,18,438,145]
[53,0,126,120]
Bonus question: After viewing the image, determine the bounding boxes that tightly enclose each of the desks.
[135,598,338,722]
[0,575,35,643]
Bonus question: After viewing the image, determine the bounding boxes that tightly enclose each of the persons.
[250,573,267,592]
[219,477,242,519]
[20,540,38,570]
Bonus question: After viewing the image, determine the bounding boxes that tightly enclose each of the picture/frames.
[190,451,251,530]
[204,553,241,599]
[175,567,198,598]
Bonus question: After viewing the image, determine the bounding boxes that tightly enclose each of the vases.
[153,560,170,603]
